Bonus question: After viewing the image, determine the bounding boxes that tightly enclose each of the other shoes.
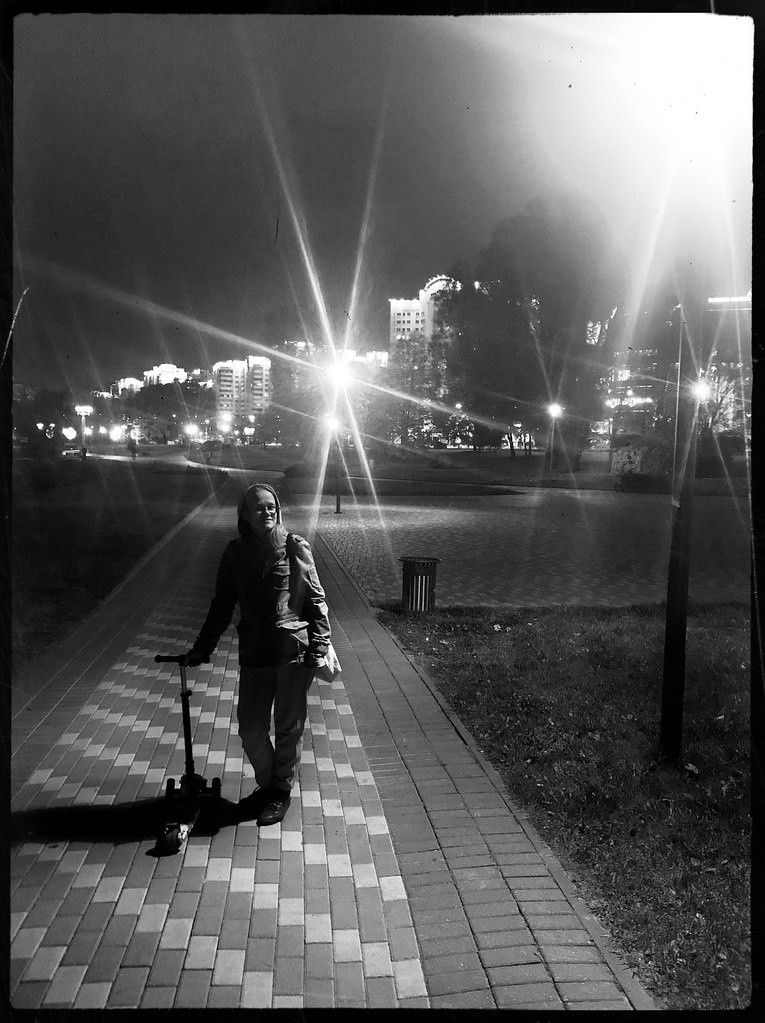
[258,797,290,825]
[238,787,275,808]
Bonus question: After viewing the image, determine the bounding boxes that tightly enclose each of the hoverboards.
[159,651,222,856]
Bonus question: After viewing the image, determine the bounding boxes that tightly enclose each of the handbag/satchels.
[312,638,342,682]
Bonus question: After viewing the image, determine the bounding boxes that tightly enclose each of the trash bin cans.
[399,557,442,614]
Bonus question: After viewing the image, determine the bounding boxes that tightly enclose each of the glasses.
[243,505,281,516]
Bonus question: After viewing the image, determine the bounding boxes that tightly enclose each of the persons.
[186,484,331,823]
[130,443,136,462]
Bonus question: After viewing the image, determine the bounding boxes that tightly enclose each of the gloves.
[182,647,204,667]
[309,643,328,658]
